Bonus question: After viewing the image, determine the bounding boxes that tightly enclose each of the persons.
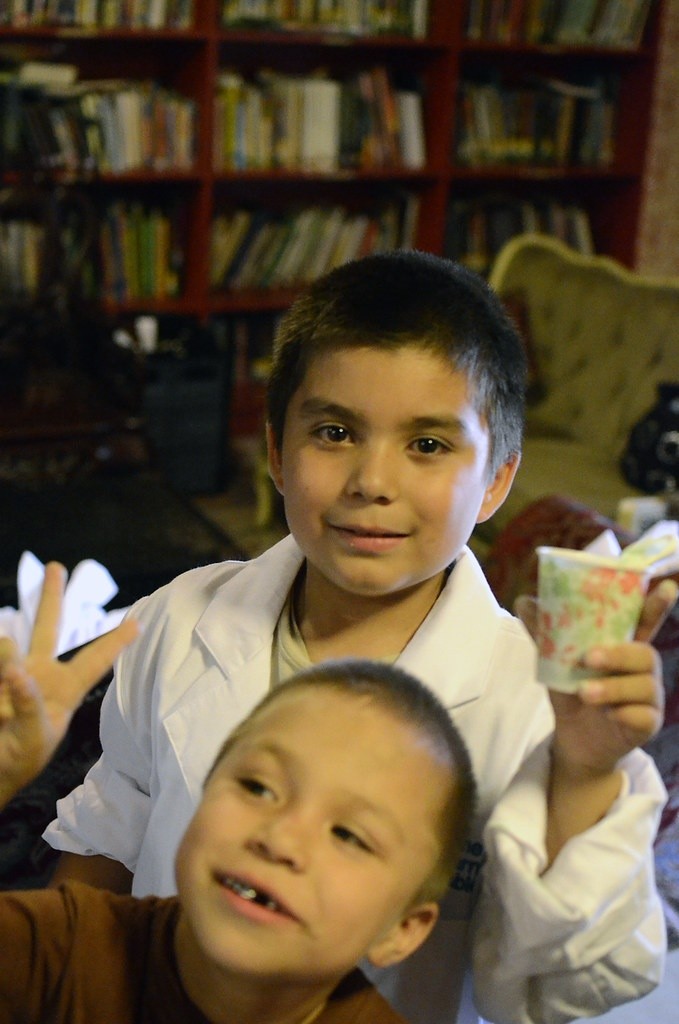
[0,562,477,1024]
[39,249,679,1024]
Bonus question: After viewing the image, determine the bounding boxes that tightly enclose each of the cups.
[535,546,652,695]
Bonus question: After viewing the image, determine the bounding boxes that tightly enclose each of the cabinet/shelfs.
[0,0,664,580]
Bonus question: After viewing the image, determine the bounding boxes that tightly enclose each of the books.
[0,0,650,301]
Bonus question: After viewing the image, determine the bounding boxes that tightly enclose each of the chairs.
[479,494,634,621]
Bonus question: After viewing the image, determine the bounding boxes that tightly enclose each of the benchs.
[252,235,679,547]
